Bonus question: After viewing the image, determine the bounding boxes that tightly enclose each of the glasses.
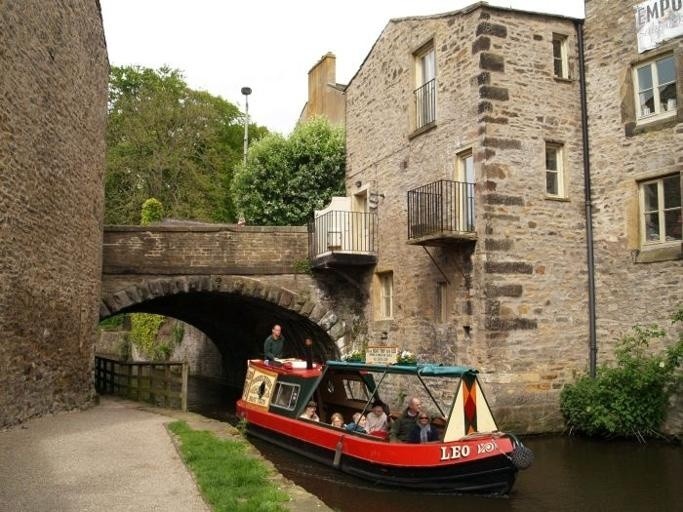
[419,417,428,419]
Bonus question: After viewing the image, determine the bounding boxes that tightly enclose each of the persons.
[331,397,439,443]
[264,324,285,360]
[300,401,320,423]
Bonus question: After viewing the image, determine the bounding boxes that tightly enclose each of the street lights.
[241,87,252,182]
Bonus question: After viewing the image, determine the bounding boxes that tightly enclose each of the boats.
[236,339,516,498]
